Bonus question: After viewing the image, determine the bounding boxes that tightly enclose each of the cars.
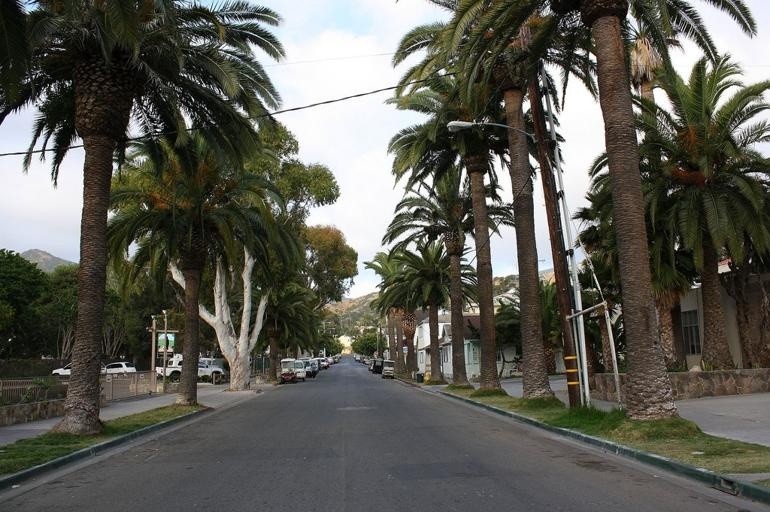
[155,353,225,384]
[280,353,341,383]
[106,362,136,373]
[354,353,394,379]
[52,362,106,374]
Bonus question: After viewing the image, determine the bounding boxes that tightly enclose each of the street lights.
[447,121,592,409]
